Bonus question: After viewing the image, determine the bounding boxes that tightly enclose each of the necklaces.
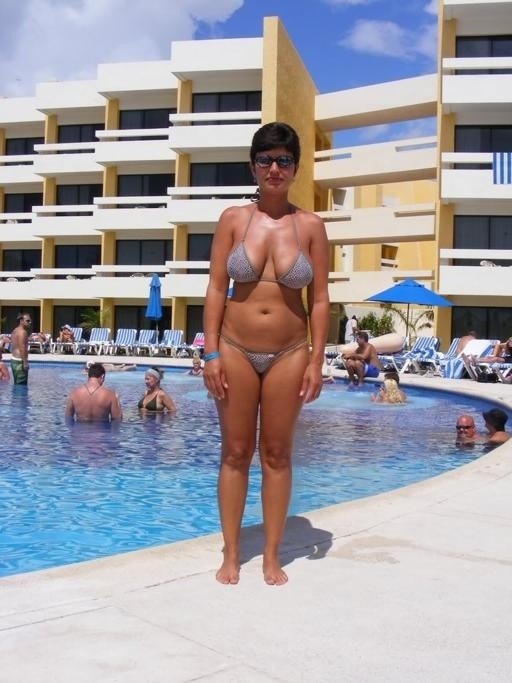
[84,383,100,400]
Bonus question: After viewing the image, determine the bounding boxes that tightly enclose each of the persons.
[202,123,331,586]
[137,368,177,413]
[456,416,479,448]
[323,315,407,405]
[482,408,510,443]
[0,312,74,384]
[65,366,122,425]
[456,330,512,366]
[186,357,204,377]
[83,363,137,374]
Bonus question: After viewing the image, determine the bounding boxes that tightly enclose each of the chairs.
[53,327,89,356]
[378,336,440,373]
[1,326,54,357]
[82,328,115,356]
[151,329,184,356]
[408,336,502,380]
[105,329,138,356]
[131,329,160,355]
[324,351,348,370]
[174,331,205,358]
[468,344,512,383]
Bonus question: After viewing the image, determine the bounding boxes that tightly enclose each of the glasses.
[456,425,469,430]
[254,156,294,169]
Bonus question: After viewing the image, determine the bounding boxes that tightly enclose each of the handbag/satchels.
[478,372,497,383]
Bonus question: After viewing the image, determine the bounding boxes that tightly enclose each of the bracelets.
[203,352,222,361]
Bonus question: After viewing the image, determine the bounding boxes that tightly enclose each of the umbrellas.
[364,279,455,337]
[226,288,234,297]
[145,274,163,344]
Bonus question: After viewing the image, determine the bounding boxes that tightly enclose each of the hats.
[484,408,508,431]
